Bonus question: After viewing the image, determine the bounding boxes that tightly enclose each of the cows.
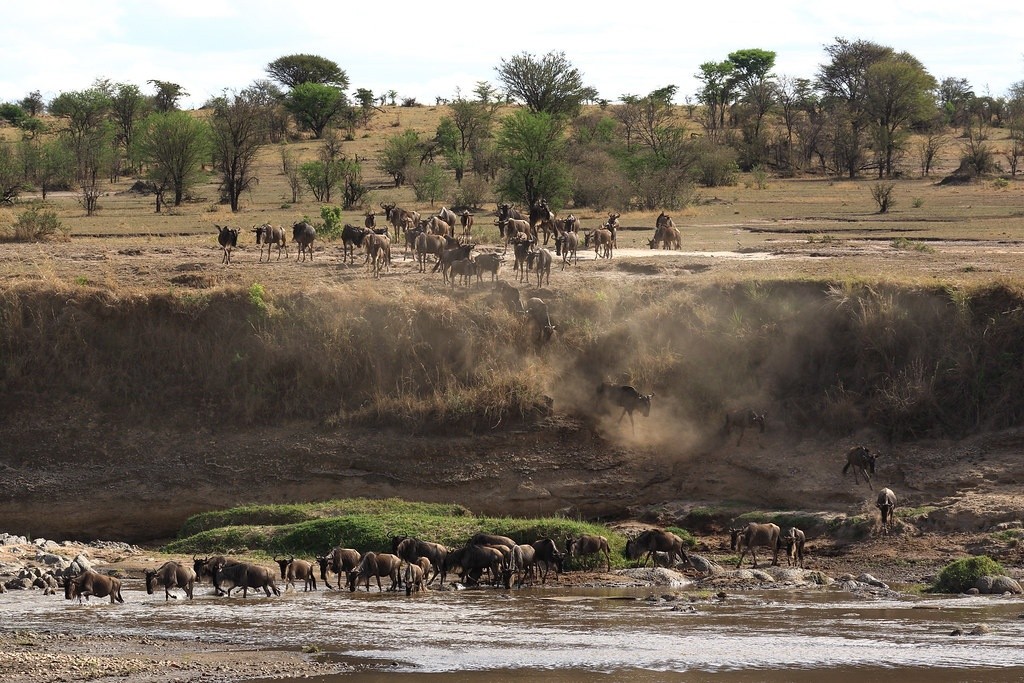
[723,406,769,449]
[143,561,197,601]
[565,534,612,572]
[626,529,685,568]
[273,553,316,591]
[349,551,403,592]
[779,528,806,568]
[595,381,654,435]
[498,280,524,318]
[526,296,555,340]
[843,445,881,492]
[387,530,565,596]
[60,571,124,604]
[646,212,683,251]
[215,202,621,287]
[874,486,897,527]
[193,552,280,597]
[728,521,782,568]
[315,546,361,589]
